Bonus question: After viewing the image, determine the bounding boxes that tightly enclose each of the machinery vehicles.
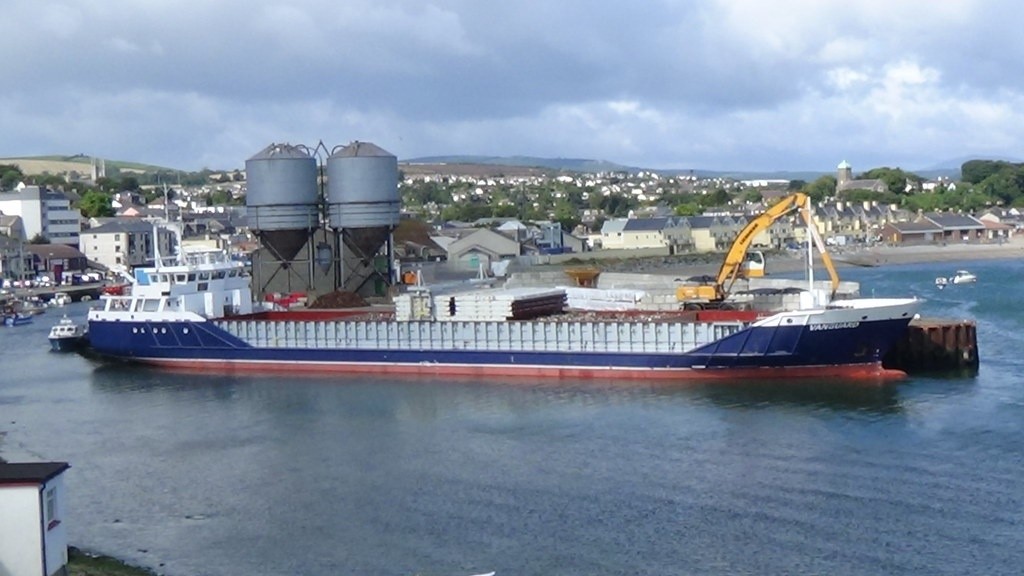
[674,190,839,312]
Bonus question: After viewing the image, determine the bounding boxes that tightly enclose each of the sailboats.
[0,240,45,315]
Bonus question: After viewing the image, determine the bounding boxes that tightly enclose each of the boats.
[949,270,978,284]
[48,317,83,352]
[0,312,33,326]
[82,217,918,379]
[51,293,71,305]
[936,277,947,288]
